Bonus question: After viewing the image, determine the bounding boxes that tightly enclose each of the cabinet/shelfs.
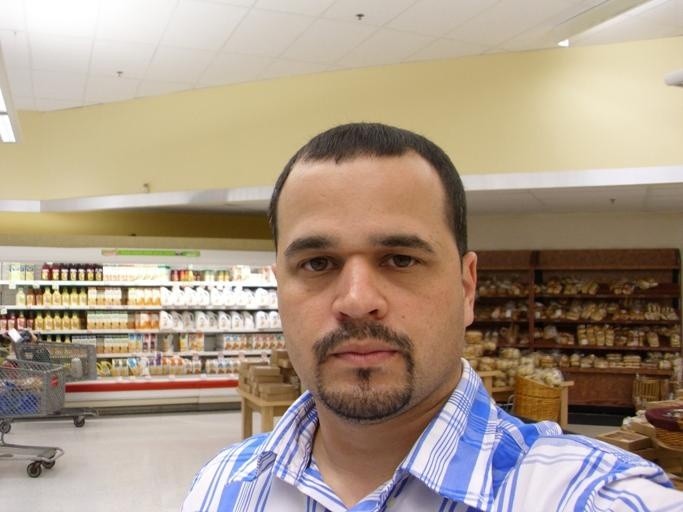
[0,247,683,442]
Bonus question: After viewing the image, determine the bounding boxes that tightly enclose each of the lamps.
[0,43,23,144]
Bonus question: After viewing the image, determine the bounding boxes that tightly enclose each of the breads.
[463,277,682,387]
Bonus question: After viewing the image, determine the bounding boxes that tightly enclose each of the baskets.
[649,425,682,476]
[509,373,562,427]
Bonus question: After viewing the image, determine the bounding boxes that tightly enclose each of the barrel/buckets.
[160,288,281,331]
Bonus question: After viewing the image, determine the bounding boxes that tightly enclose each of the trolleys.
[0,329,99,477]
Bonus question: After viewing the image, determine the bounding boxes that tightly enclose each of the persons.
[178,122,683,511]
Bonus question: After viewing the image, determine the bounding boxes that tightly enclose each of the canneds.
[171,269,233,281]
